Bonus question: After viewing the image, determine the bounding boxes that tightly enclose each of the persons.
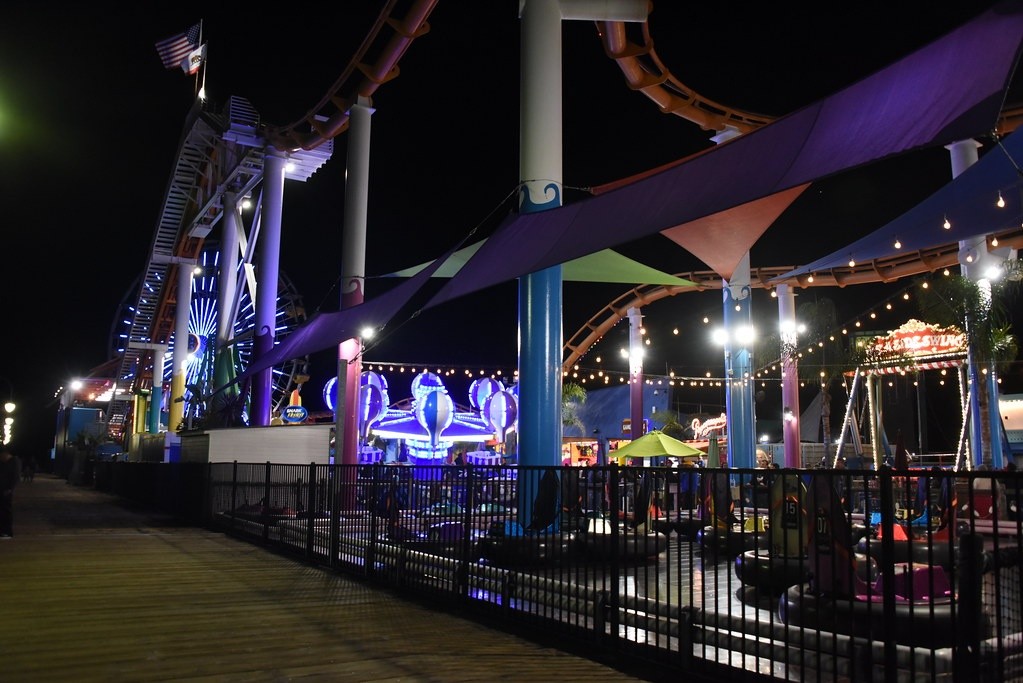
[582,461,600,510]
[24,463,35,482]
[973,465,999,488]
[678,460,686,468]
[746,460,780,487]
[698,458,704,467]
[0,449,21,539]
[454,453,464,465]
[722,462,728,468]
[379,461,407,487]
[502,458,506,466]
[651,459,679,490]
[836,458,854,504]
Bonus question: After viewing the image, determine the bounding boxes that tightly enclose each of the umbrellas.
[706,431,723,516]
[607,426,705,466]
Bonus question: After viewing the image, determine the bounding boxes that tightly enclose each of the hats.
[0,445,9,451]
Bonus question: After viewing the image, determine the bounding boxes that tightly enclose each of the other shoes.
[0,533,12,539]
